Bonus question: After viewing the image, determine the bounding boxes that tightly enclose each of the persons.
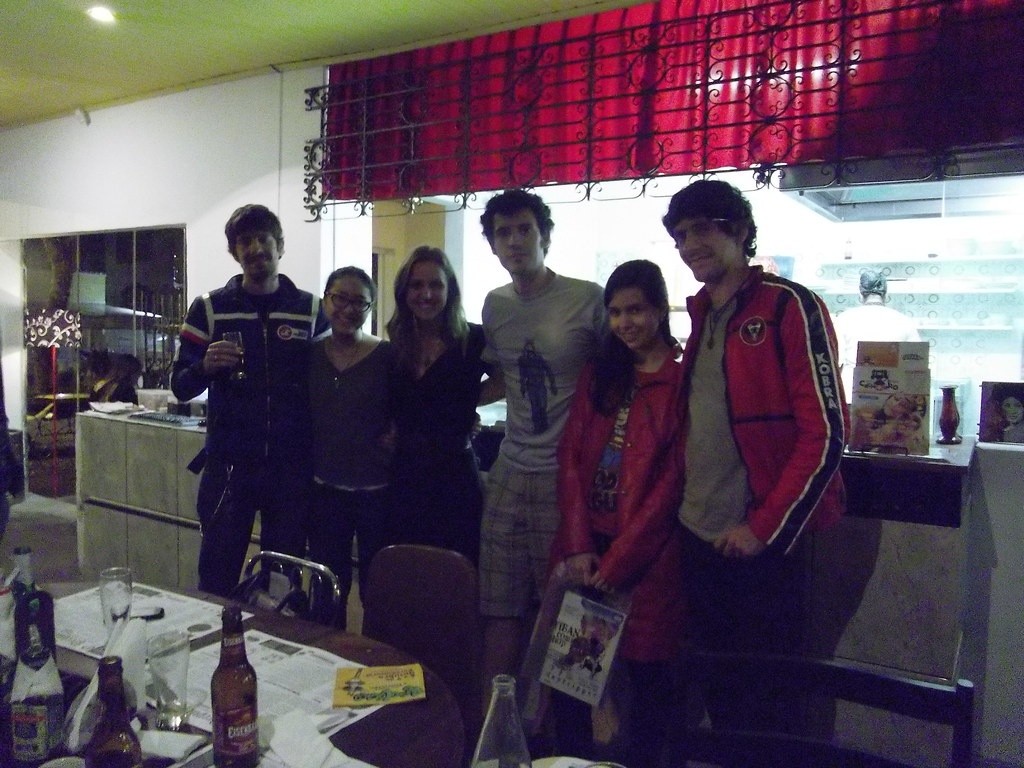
[291,268,389,630]
[386,245,502,563]
[853,394,918,446]
[835,271,922,407]
[990,383,1024,443]
[545,260,687,768]
[663,181,852,740]
[479,189,607,758]
[171,205,330,596]
[91,354,142,405]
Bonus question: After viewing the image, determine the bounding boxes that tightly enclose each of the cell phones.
[132,607,165,619]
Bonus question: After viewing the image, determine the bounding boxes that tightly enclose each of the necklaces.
[332,336,363,381]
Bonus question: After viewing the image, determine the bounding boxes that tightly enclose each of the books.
[847,342,930,455]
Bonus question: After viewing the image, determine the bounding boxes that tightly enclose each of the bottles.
[470,672,533,768]
[212,605,259,768]
[935,384,963,444]
[85,655,144,768]
[11,546,66,762]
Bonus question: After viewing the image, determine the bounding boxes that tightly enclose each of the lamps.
[24,308,84,500]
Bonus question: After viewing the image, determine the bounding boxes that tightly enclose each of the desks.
[0,581,467,768]
[35,394,91,435]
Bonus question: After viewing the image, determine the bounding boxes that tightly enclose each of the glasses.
[673,218,731,247]
[324,291,375,314]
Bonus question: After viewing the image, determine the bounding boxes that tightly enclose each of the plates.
[532,756,611,768]
[38,756,86,768]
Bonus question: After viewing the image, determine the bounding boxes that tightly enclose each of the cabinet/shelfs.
[820,256,1024,330]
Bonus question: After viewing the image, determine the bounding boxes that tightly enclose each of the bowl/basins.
[135,389,178,413]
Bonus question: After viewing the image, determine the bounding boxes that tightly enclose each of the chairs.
[660,637,980,768]
[362,542,483,738]
[240,550,343,627]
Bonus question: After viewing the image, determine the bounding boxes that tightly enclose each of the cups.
[99,566,132,638]
[146,630,191,732]
[222,331,247,381]
